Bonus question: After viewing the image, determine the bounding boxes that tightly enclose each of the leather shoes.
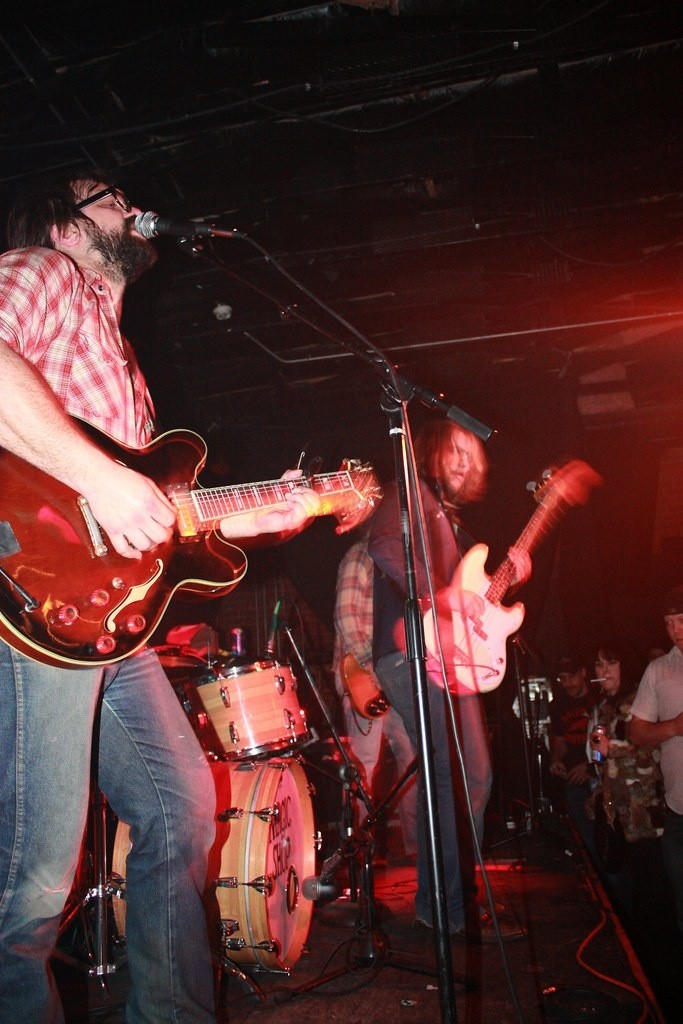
[413,899,528,944]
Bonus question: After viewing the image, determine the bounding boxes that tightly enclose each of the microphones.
[301,877,341,901]
[263,600,282,659]
[135,213,247,241]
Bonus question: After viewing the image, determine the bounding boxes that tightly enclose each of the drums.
[178,656,313,763]
[109,756,319,972]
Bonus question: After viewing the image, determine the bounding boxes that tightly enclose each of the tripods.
[279,618,481,1005]
[52,778,129,1002]
[482,635,588,879]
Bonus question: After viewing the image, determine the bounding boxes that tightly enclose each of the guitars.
[392,458,593,700]
[340,651,394,720]
[0,412,387,671]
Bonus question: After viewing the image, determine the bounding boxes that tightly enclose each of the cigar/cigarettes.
[590,678,606,682]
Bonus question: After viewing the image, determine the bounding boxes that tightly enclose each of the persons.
[549,584,683,932]
[0,178,321,1023]
[333,416,531,943]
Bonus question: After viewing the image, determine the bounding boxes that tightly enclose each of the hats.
[556,650,582,675]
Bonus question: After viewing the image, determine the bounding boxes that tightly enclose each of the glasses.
[77,186,132,213]
